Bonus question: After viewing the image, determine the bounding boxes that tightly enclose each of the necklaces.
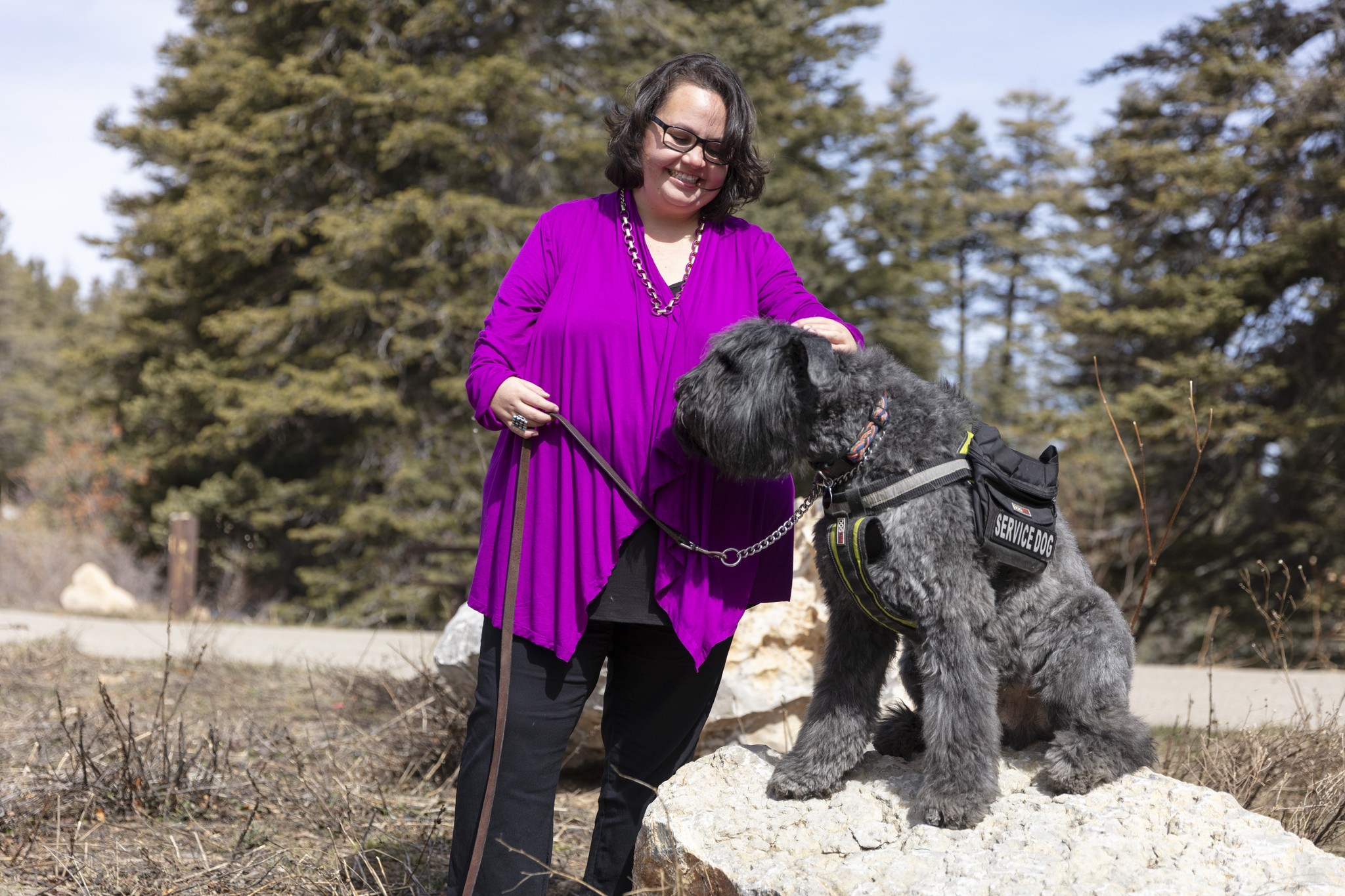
[618,185,710,317]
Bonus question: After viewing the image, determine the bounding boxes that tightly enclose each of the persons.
[440,53,868,896]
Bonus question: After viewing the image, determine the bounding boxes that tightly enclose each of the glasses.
[648,111,739,167]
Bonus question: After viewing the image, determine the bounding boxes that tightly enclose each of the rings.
[511,415,528,431]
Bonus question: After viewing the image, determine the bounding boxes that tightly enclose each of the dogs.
[670,312,1167,833]
